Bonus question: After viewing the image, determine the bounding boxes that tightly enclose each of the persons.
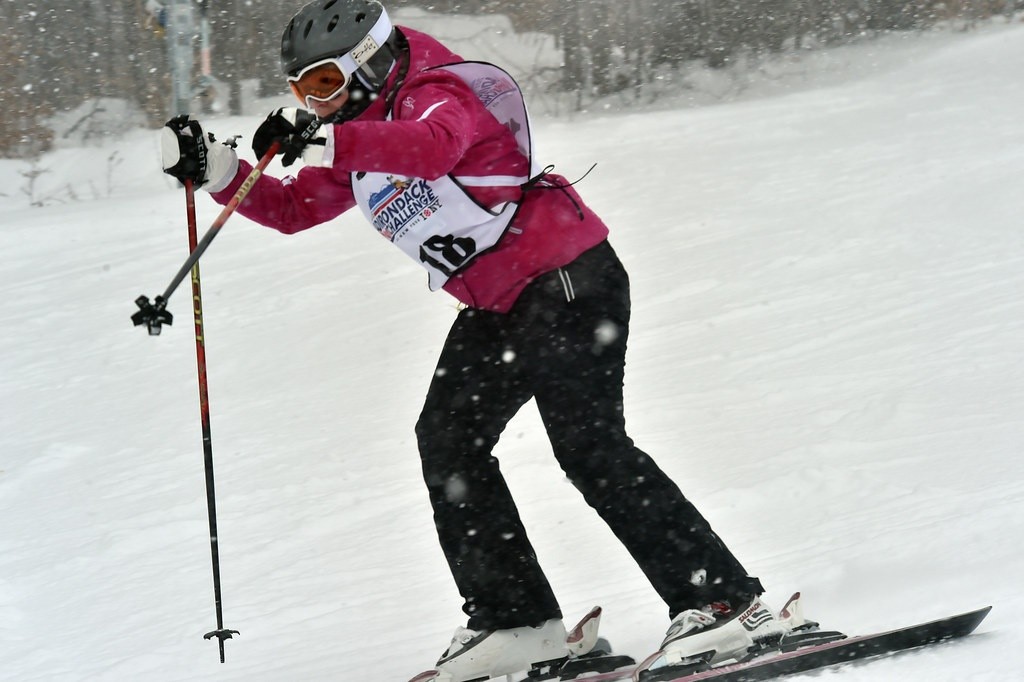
[159,0,786,681]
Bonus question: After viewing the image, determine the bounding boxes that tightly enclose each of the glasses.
[285,57,350,110]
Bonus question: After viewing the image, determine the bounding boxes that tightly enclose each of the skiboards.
[406,591,993,681]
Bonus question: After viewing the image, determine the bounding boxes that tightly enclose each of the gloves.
[161,114,209,192]
[252,105,320,167]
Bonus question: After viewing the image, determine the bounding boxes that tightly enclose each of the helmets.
[280,0,398,125]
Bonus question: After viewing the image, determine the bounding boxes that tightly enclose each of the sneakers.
[632,591,796,680]
[409,617,572,681]
